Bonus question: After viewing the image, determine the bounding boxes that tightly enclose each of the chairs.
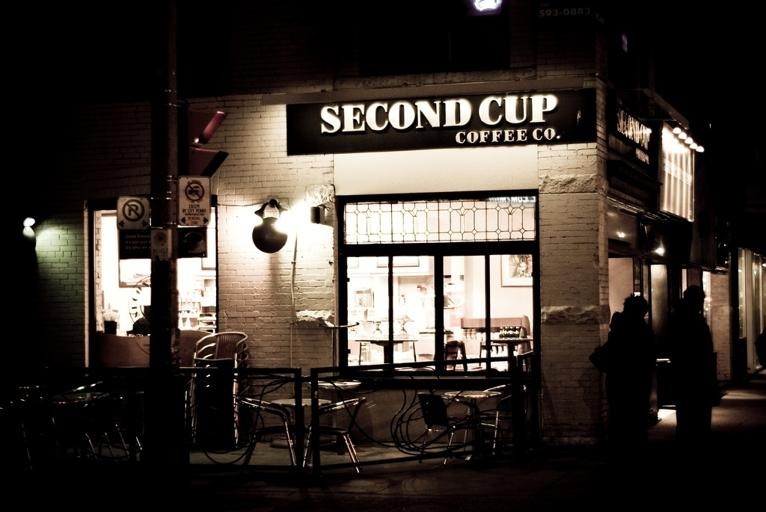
[186,330,250,453]
[444,335,534,371]
[235,369,527,475]
[0,354,149,473]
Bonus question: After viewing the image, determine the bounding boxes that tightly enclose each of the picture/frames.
[500,255,532,288]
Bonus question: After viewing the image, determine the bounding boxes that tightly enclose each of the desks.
[354,336,420,365]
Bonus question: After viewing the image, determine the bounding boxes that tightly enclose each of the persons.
[663,286,719,469]
[606,295,659,472]
